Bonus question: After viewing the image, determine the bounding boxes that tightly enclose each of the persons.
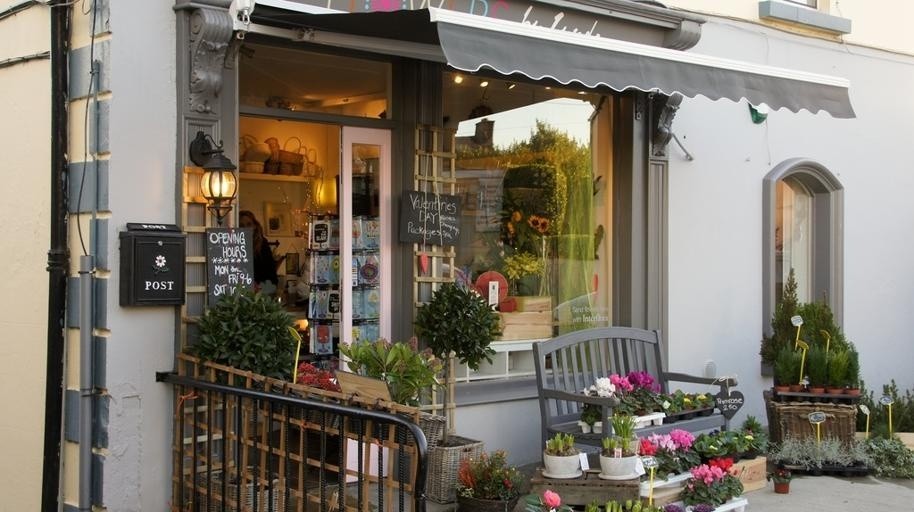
[238,209,279,300]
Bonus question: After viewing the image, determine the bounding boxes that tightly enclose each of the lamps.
[189,129,237,225]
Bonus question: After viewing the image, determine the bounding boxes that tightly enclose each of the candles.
[757,265,861,395]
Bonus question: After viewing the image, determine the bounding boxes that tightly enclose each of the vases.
[774,482,789,493]
[517,272,542,297]
[457,490,519,510]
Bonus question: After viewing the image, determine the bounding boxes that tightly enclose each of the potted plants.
[411,281,504,503]
[542,432,581,477]
[190,286,295,405]
[338,337,445,447]
[597,415,645,480]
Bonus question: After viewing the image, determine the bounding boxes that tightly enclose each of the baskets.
[239,135,315,176]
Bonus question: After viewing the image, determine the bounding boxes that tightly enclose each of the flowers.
[768,465,791,483]
[640,416,764,487]
[664,504,684,512]
[293,360,343,394]
[524,487,572,511]
[682,462,743,506]
[483,211,551,277]
[581,368,718,435]
[693,500,715,512]
[453,448,524,499]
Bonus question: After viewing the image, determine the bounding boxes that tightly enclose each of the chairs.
[532,327,738,461]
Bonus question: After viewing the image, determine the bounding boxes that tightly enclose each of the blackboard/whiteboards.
[399,190,460,246]
[206,226,254,311]
